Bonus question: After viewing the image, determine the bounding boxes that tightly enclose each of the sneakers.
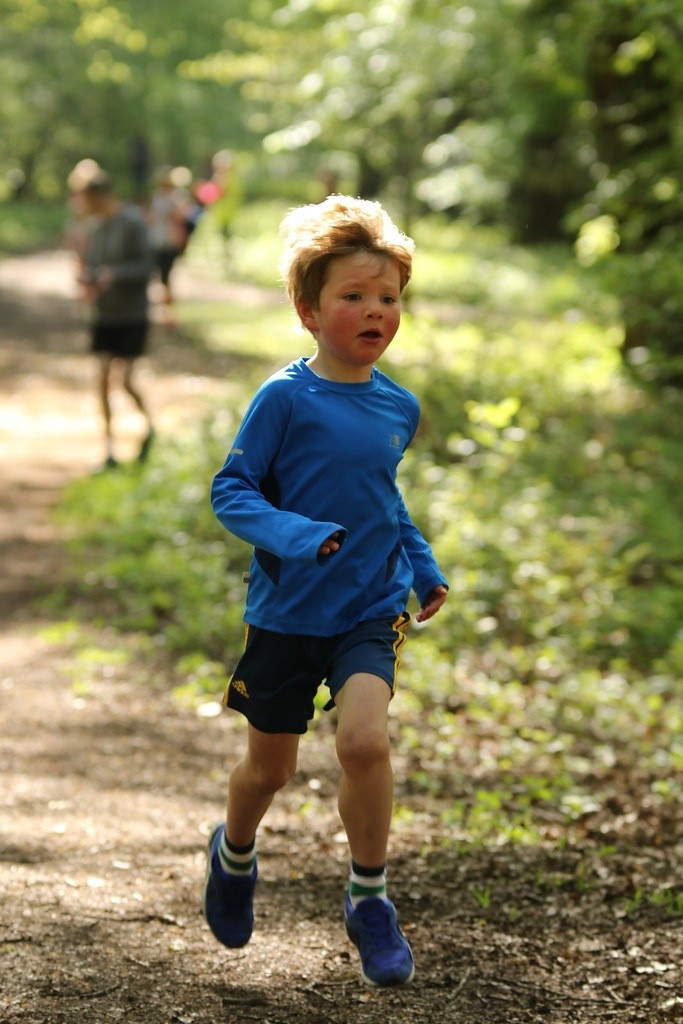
[201,823,259,949]
[343,894,415,989]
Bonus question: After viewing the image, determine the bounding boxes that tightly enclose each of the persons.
[203,192,454,991]
[65,158,157,481]
[160,161,206,312]
